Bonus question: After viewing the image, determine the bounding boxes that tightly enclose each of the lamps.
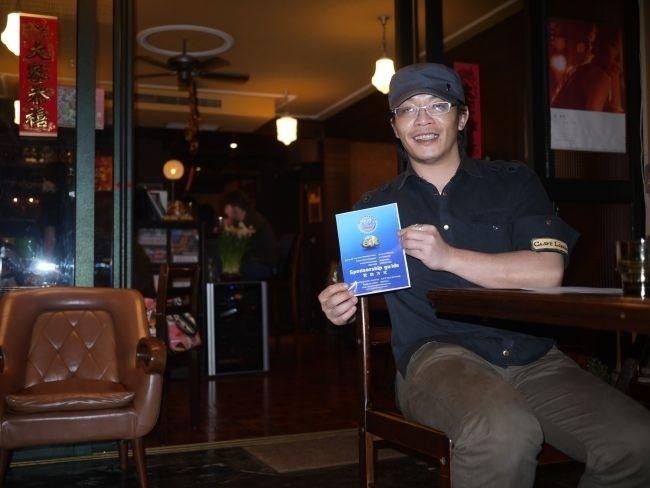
[162,159,185,201]
[369,15,396,95]
[273,89,299,146]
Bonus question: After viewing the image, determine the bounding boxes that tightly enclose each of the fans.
[135,38,250,86]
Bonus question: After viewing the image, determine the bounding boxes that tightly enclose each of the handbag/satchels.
[166,312,203,353]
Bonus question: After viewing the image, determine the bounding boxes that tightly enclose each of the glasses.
[392,101,456,119]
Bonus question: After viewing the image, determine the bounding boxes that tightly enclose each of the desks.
[425,287,649,412]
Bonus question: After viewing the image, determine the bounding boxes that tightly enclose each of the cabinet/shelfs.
[203,279,270,380]
[136,219,206,382]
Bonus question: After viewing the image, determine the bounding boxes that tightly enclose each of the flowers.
[211,203,257,272]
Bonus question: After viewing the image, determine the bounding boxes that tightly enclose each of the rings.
[414,223,423,231]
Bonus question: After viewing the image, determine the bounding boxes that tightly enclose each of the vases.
[220,272,242,282]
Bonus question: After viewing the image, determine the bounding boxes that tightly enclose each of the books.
[519,286,622,294]
[335,202,411,298]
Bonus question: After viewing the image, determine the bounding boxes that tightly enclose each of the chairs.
[0,284,167,487]
[155,262,202,443]
[354,294,572,487]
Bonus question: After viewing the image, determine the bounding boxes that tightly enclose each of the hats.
[389,63,464,108]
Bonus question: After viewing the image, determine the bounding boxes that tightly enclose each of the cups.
[615,236,650,300]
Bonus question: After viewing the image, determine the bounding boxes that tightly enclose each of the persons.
[215,190,279,302]
[39,176,75,257]
[317,61,650,488]
